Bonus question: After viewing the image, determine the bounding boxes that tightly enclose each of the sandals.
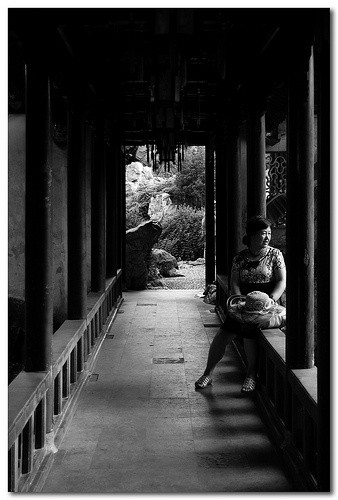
[195,374,213,388]
[242,378,256,393]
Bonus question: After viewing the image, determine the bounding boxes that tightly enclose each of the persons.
[193,216,286,392]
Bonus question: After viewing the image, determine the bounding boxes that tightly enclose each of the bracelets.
[269,294,276,302]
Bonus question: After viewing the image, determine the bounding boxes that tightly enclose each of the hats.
[227,291,287,317]
